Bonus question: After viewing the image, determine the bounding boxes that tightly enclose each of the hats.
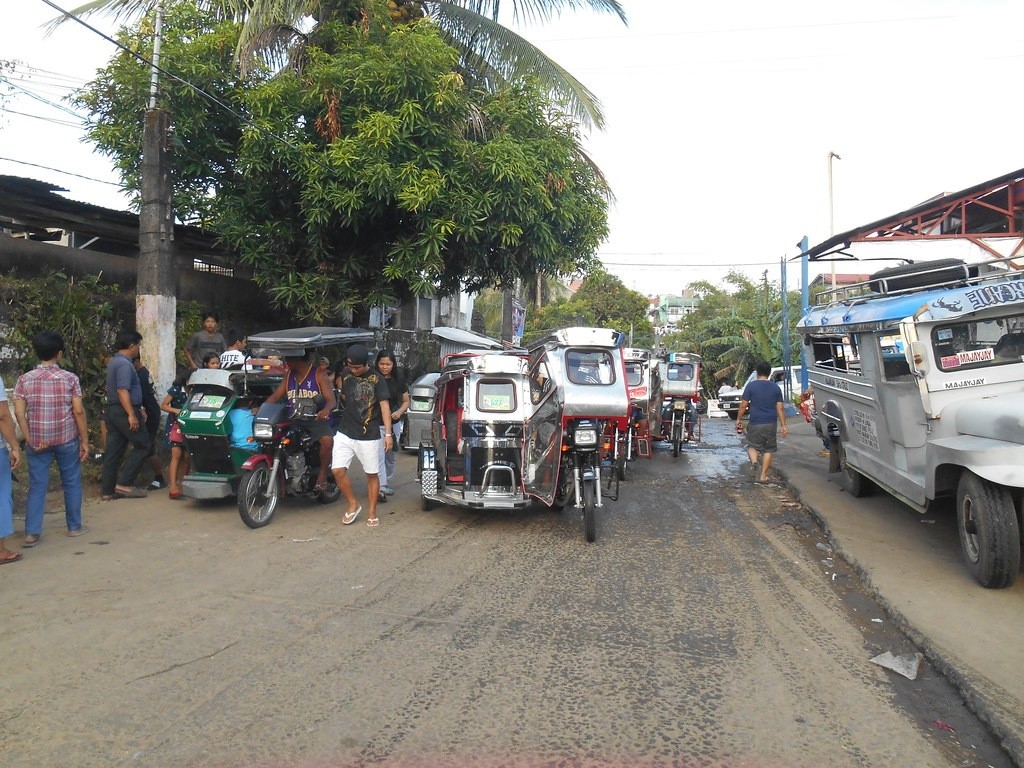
[345,345,368,364]
[319,357,329,365]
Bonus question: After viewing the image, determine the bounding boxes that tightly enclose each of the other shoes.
[689,434,699,441]
[385,486,394,496]
[169,488,183,499]
[377,492,387,502]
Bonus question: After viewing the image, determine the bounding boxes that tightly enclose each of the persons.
[662,368,697,439]
[374,349,410,503]
[0,376,23,564]
[331,344,393,527]
[718,379,735,394]
[736,362,788,484]
[181,313,248,371]
[780,373,784,381]
[543,352,602,393]
[97,330,168,500]
[253,349,336,492]
[14,332,91,548]
[160,367,199,499]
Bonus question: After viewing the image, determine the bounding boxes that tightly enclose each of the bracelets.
[385,434,392,437]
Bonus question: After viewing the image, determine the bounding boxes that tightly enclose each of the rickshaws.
[179,325,375,528]
[401,324,709,544]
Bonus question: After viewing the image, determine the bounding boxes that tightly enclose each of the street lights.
[825,152,841,305]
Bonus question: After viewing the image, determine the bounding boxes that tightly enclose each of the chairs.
[994,333,1024,359]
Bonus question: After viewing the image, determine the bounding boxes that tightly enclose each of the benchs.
[834,358,914,383]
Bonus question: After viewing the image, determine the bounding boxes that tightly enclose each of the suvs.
[717,365,810,420]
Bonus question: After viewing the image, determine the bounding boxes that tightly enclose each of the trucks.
[795,254,1023,588]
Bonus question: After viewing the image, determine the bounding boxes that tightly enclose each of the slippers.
[760,477,769,481]
[116,485,147,498]
[0,549,23,564]
[23,535,41,547]
[102,492,116,500]
[147,481,167,491]
[367,515,380,526]
[343,504,362,525]
[314,481,327,492]
[68,525,88,537]
[751,462,758,479]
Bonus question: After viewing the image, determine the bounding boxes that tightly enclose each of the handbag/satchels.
[169,423,184,443]
